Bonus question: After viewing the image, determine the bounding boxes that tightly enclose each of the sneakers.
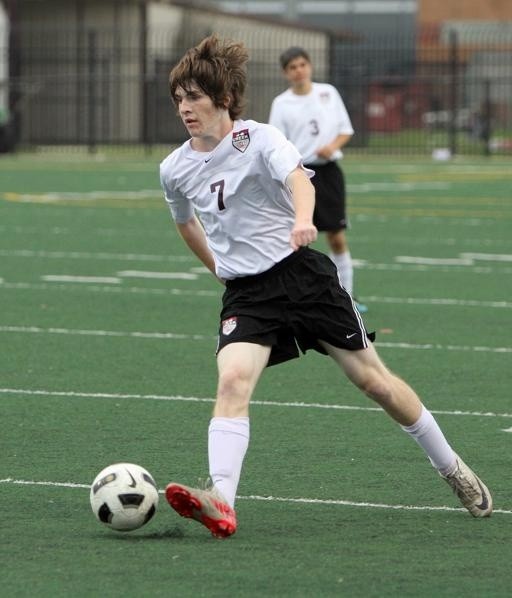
[163,480,239,539]
[427,450,493,519]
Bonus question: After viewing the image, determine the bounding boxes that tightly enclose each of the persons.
[268,48,366,312]
[160,36,493,538]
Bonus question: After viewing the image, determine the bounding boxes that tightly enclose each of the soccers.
[89,464,160,530]
[432,147,452,164]
